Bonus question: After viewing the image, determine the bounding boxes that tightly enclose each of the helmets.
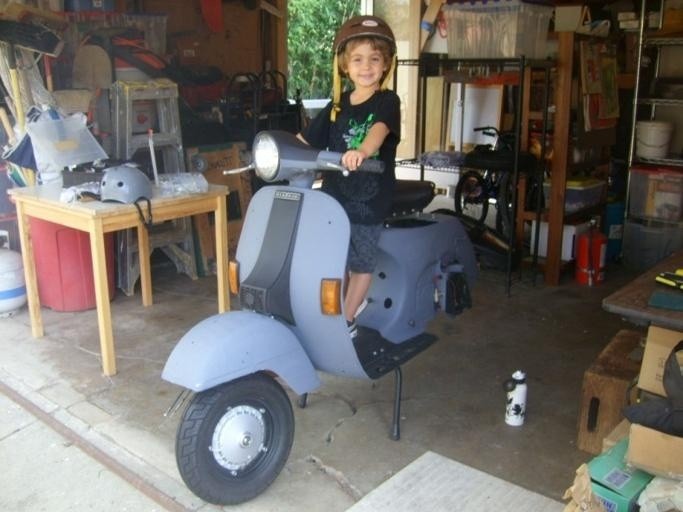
[98,164,154,206]
[331,14,396,78]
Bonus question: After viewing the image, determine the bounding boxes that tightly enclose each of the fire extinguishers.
[574,216,608,287]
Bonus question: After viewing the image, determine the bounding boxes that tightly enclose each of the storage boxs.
[438,0,555,63]
[577,326,683,510]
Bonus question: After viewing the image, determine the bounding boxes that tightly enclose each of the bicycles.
[455,127,513,222]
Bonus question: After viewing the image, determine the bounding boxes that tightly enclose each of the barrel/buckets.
[634,120,675,159]
[27,215,116,311]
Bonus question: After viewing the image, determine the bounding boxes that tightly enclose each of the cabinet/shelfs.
[521,2,683,290]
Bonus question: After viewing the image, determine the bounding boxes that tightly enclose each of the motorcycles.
[161,133,478,505]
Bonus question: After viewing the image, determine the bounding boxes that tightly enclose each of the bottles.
[504,369,528,426]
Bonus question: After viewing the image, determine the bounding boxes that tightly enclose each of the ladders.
[109,78,199,296]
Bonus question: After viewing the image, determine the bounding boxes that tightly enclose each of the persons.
[294,15,401,340]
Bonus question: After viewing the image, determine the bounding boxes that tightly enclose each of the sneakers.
[344,297,368,339]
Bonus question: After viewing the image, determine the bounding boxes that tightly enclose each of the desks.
[7,174,234,380]
[599,249,681,328]
